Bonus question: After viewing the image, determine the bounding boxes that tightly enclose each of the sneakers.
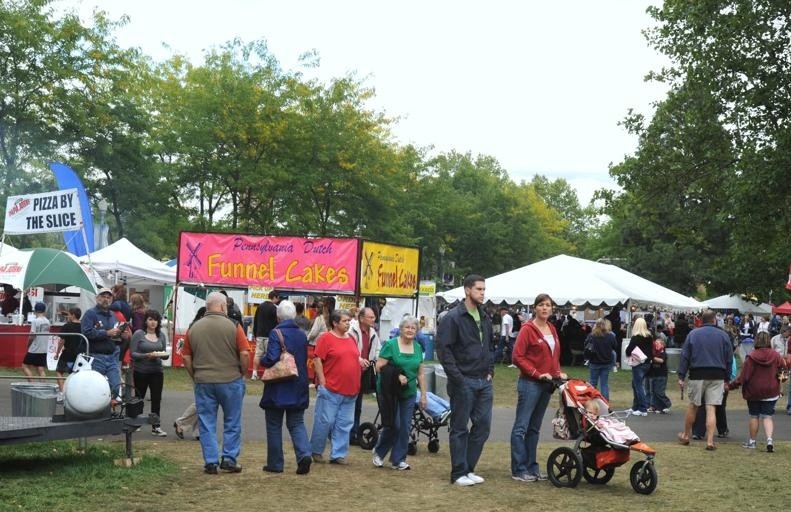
[370,446,385,469]
[678,430,690,445]
[765,437,775,453]
[390,461,411,471]
[717,429,729,438]
[151,427,168,437]
[662,407,671,414]
[531,470,550,481]
[705,442,716,451]
[512,469,536,483]
[654,410,662,415]
[740,437,758,450]
[624,408,633,413]
[451,472,473,488]
[632,410,647,417]
[692,434,703,440]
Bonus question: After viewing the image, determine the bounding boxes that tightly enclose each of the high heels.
[174,419,185,441]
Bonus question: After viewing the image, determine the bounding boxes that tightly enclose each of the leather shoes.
[203,463,219,475]
[466,470,484,485]
[310,449,326,464]
[296,455,312,474]
[262,463,283,473]
[220,461,243,473]
[329,455,352,467]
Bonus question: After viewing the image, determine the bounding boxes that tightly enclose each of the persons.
[311,309,360,464]
[173,306,207,440]
[498,308,514,365]
[586,321,617,372]
[551,312,583,351]
[111,310,133,387]
[691,318,729,440]
[626,316,652,416]
[585,319,617,400]
[294,304,312,331]
[586,401,641,445]
[585,348,617,373]
[652,306,791,336]
[253,292,280,381]
[182,291,251,472]
[734,333,791,452]
[308,298,331,387]
[22,305,51,379]
[109,286,131,321]
[437,275,496,485]
[512,305,527,338]
[348,307,382,438]
[677,309,732,452]
[257,298,312,474]
[129,310,169,436]
[651,337,672,414]
[54,309,87,402]
[130,295,145,331]
[373,318,427,470]
[81,290,127,410]
[0,285,20,316]
[512,293,568,482]
[769,328,791,362]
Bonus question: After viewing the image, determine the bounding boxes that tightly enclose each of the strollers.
[540,377,658,496]
[357,360,448,455]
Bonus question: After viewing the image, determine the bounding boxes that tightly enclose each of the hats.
[96,287,113,296]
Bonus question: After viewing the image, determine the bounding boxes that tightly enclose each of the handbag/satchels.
[586,335,598,363]
[261,326,298,383]
